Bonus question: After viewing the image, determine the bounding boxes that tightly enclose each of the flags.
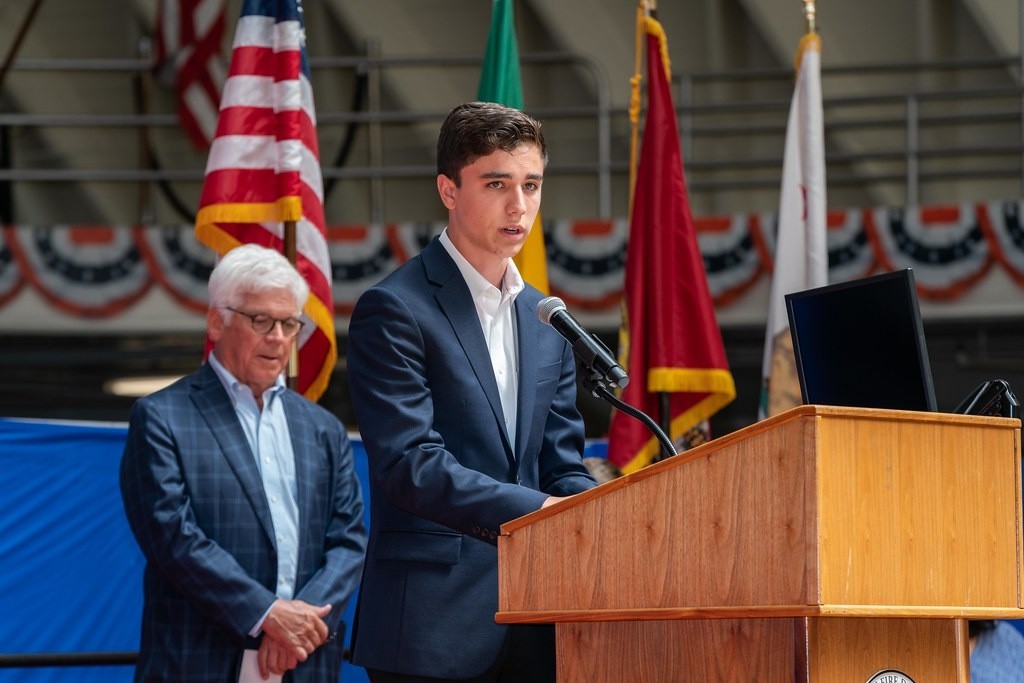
[477,0,550,298]
[757,32,827,424]
[195,1,337,404]
[607,2,736,476]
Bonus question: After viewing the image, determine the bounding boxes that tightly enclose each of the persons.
[969,620,1024,683]
[347,102,605,683]
[120,244,366,683]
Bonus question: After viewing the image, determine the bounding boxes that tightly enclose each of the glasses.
[225,306,305,337]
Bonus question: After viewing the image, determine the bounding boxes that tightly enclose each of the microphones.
[537,296,630,389]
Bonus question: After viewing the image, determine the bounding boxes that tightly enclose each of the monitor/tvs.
[784,268,940,414]
